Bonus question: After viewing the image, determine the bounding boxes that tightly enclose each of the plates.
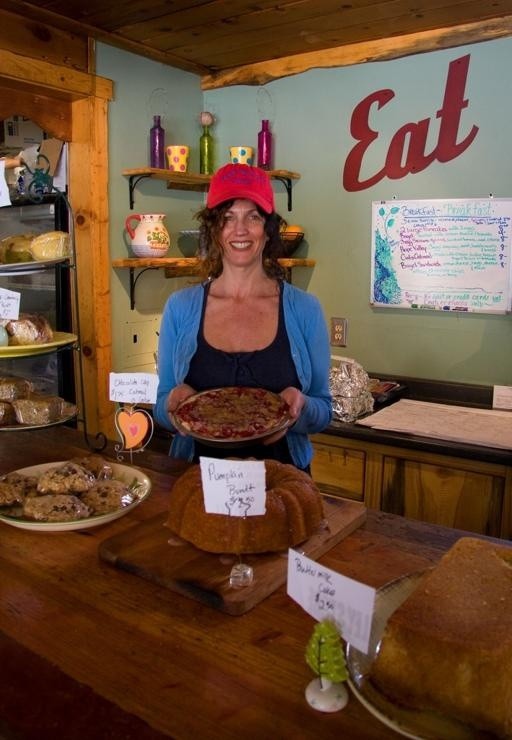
[0,401,81,433]
[345,563,491,740]
[0,460,151,535]
[0,331,79,359]
[0,255,70,271]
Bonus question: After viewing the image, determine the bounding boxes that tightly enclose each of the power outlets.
[329,317,347,347]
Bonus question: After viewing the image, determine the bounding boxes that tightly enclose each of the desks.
[0,426,512,740]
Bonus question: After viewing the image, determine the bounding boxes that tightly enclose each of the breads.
[22,493,91,524]
[162,457,329,557]
[0,372,32,404]
[12,393,65,426]
[365,534,512,737]
[82,477,138,516]
[36,462,96,493]
[1,314,55,345]
[0,469,38,510]
[1,402,10,428]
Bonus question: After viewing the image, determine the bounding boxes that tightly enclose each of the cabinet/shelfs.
[377,445,510,541]
[111,141,318,310]
[303,431,375,509]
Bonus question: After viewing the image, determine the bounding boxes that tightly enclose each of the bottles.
[125,214,171,258]
[150,113,165,168]
[200,125,214,174]
[258,120,272,167]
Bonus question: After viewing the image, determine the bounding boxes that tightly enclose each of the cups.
[166,145,190,173]
[231,146,253,166]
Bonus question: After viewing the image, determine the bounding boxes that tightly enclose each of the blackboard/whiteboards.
[370,198,512,316]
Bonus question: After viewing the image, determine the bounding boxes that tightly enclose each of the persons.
[152,165,333,481]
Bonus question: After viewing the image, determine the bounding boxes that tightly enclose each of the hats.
[206,164,274,214]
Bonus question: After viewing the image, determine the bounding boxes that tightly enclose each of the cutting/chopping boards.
[99,493,367,616]
